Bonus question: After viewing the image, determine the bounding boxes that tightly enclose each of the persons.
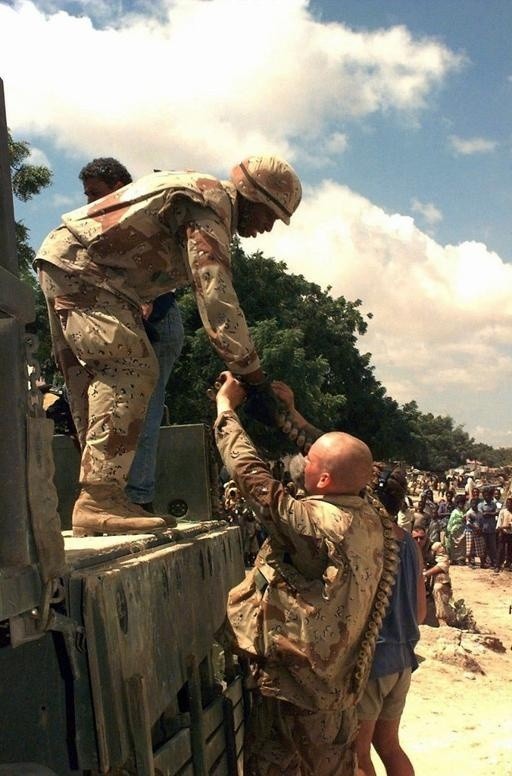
[352,474,427,776]
[224,458,512,571]
[79,157,184,513]
[213,370,400,776]
[412,526,453,626]
[31,153,302,537]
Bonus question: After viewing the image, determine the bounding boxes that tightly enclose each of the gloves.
[240,380,277,436]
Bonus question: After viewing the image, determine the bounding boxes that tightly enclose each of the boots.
[72,487,177,538]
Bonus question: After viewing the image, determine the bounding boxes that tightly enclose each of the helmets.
[231,155,302,225]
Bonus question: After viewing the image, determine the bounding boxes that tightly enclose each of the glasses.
[414,537,425,541]
